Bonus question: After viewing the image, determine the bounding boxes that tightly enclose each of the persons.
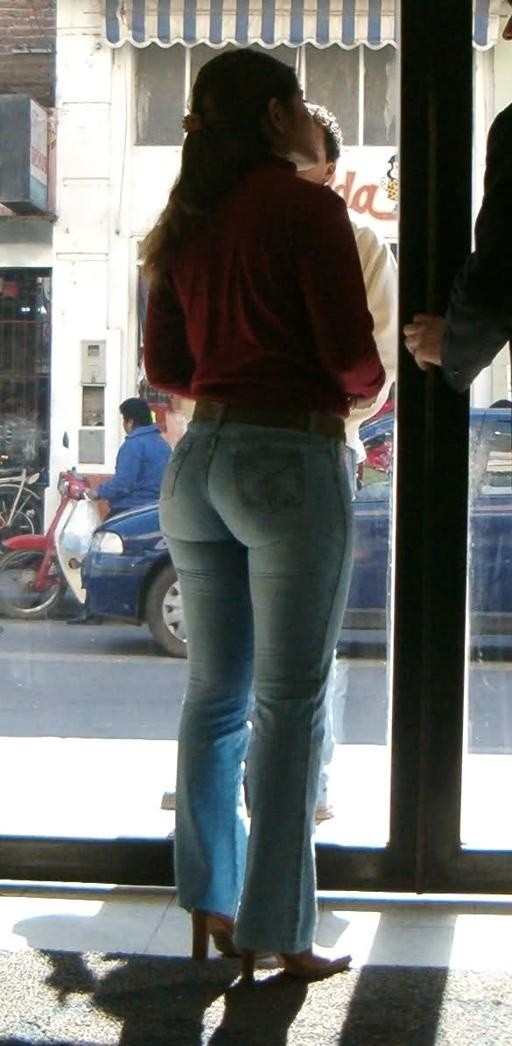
[405,9,509,394]
[161,101,399,821]
[67,397,172,625]
[140,48,381,983]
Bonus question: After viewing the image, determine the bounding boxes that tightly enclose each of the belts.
[190,397,347,437]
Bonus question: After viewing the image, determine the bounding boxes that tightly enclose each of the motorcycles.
[0,453,43,566]
[0,462,96,620]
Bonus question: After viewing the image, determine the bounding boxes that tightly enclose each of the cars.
[79,407,512,665]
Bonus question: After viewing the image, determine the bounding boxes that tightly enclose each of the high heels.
[239,934,355,979]
[187,908,243,965]
